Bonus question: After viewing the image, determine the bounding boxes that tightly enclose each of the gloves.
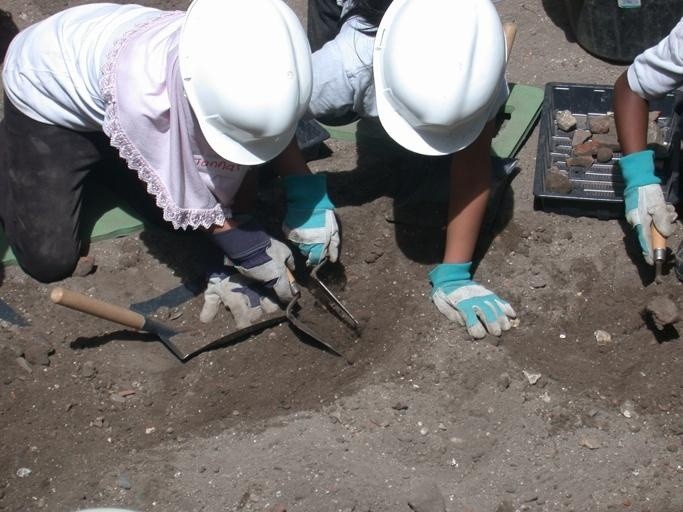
[425,261,516,340]
[277,172,339,272]
[200,244,281,330]
[617,151,673,266]
[210,212,297,304]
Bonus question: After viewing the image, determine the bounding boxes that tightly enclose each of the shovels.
[272,256,360,356]
[51,273,288,363]
[393,22,518,230]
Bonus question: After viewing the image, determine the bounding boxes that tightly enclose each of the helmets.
[372,1,507,156]
[178,1,313,167]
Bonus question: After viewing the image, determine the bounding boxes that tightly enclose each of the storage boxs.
[532,81,681,205]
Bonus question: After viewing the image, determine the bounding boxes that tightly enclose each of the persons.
[0,0,314,332]
[613,16,683,266]
[280,0,517,339]
